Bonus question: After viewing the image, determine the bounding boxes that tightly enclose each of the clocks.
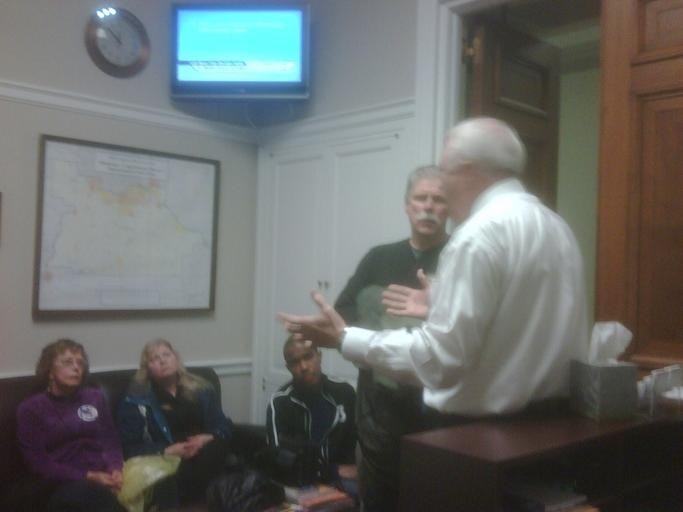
[84,7,150,79]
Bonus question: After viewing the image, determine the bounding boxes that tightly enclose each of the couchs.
[0,366,266,511]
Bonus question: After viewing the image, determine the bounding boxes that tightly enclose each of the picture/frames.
[29,133,220,319]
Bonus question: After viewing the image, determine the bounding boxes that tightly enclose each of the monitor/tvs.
[170,4,307,94]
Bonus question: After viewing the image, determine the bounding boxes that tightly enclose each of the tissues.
[573,322,638,412]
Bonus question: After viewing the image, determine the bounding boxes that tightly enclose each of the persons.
[14,337,125,512]
[334,163,453,512]
[277,113,594,434]
[116,339,270,512]
[264,333,356,512]
